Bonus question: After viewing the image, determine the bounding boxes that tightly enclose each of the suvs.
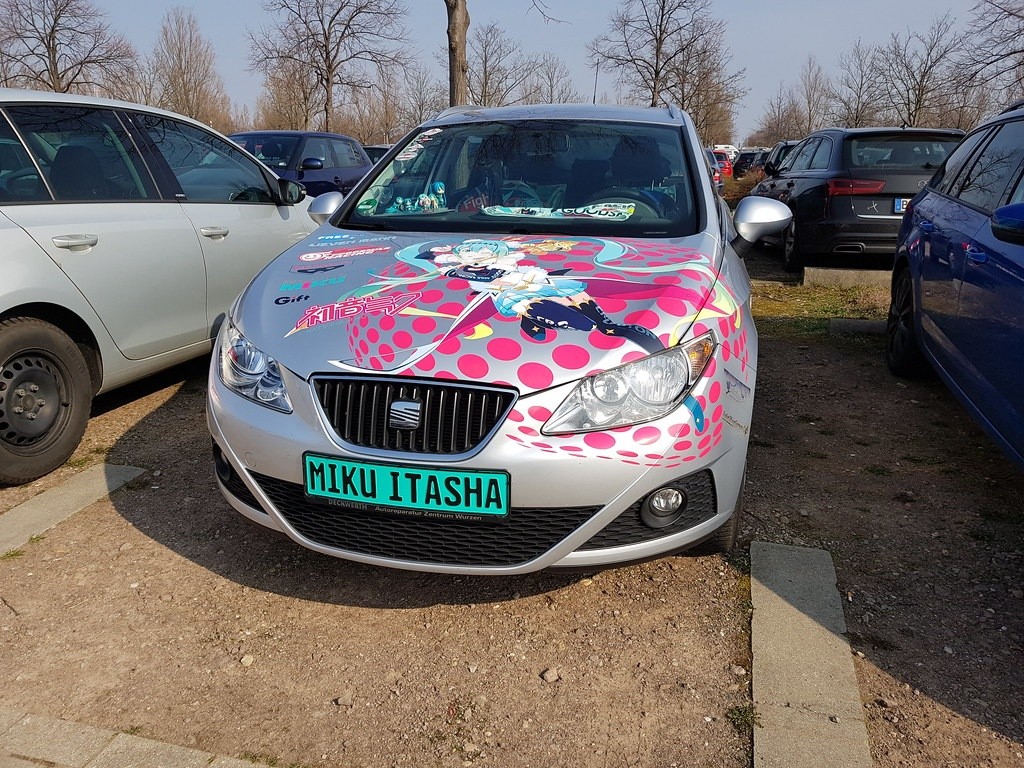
[0,89,395,485]
[885,99,1024,467]
[764,139,802,175]
[206,104,793,576]
[757,128,968,271]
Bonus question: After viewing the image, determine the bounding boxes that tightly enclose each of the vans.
[709,145,739,155]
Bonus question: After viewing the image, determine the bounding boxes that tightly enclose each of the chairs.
[607,134,675,216]
[304,143,323,160]
[261,143,280,159]
[891,147,915,161]
[50,145,108,199]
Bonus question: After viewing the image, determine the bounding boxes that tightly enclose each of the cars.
[704,147,773,206]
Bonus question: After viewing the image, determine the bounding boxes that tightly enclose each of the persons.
[405,182,446,212]
